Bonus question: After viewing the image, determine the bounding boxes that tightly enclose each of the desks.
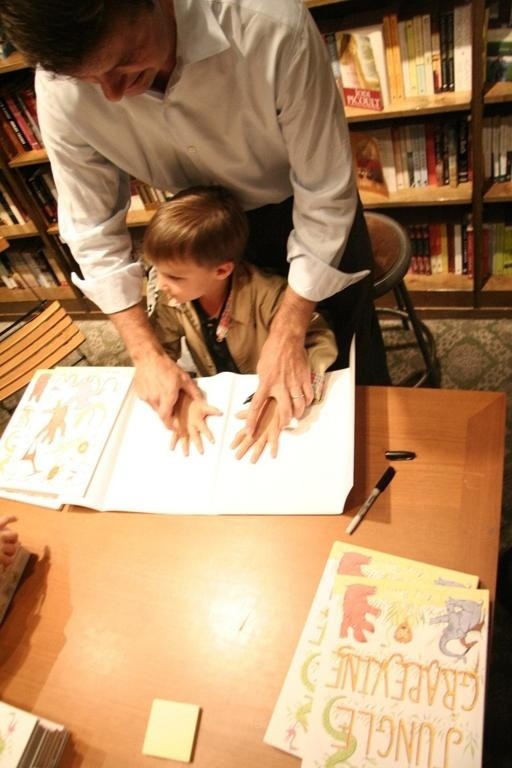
[1,366,506,766]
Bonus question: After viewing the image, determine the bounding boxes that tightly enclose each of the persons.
[0,1,390,433]
[0,514,18,572]
[147,187,340,465]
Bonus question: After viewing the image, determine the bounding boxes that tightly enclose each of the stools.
[361,211,440,388]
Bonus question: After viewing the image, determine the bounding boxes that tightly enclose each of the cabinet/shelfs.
[1,0,512,318]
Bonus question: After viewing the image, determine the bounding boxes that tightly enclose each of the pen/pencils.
[345,466,395,535]
[243,392,255,404]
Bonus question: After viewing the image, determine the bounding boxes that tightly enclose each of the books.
[320,16,512,274]
[2,39,175,290]
[1,700,70,768]
[0,544,32,627]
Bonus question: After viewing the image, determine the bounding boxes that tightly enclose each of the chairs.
[1,238,88,415]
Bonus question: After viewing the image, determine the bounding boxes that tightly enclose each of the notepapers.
[142,698,201,763]
